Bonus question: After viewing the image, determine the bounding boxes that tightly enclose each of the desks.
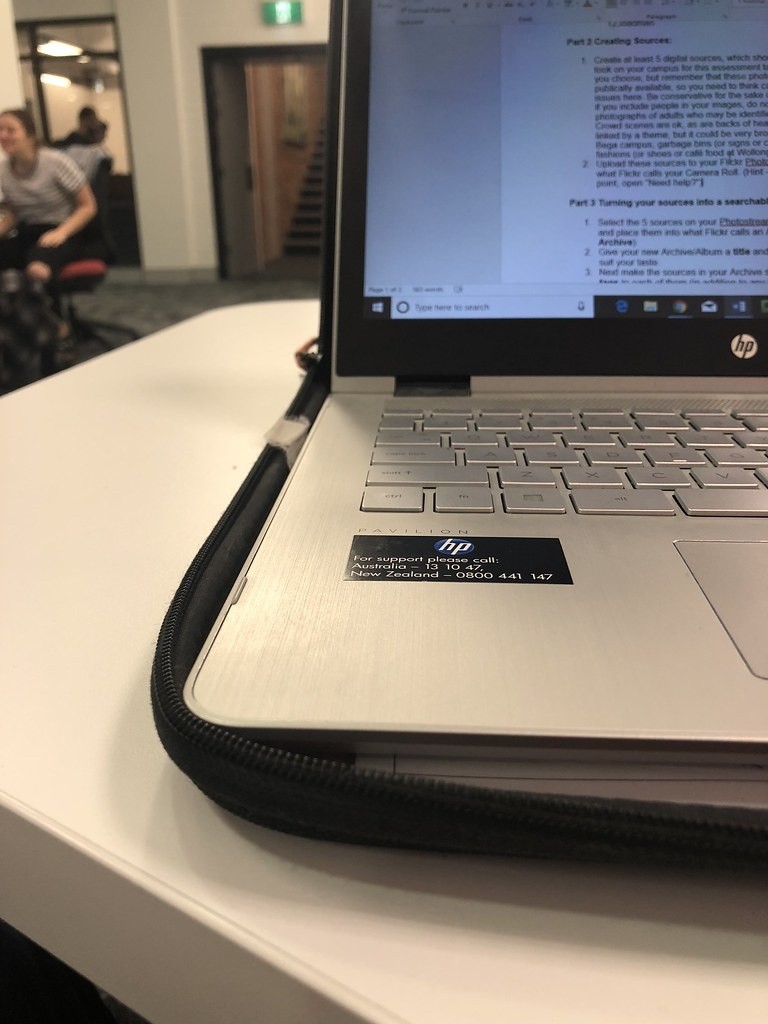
[0,296,768,1024]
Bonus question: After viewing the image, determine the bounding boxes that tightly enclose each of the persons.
[56,107,124,262]
[0,108,100,350]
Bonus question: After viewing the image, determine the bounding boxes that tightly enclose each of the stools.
[41,259,144,380]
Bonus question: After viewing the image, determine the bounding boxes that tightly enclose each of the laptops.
[184,0,768,771]
